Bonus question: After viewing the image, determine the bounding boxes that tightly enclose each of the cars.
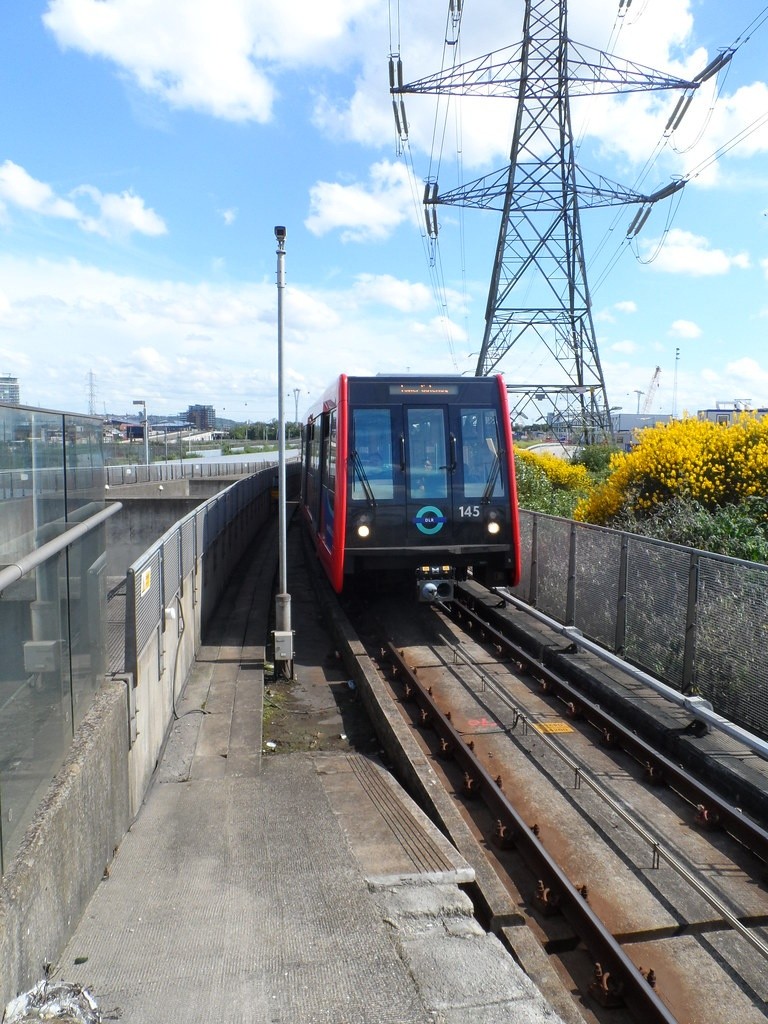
[544,437,556,443]
[556,437,568,443]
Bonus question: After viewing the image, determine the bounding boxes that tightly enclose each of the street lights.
[272,224,295,682]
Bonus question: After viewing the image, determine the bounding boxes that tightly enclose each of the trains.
[298,372,522,602]
